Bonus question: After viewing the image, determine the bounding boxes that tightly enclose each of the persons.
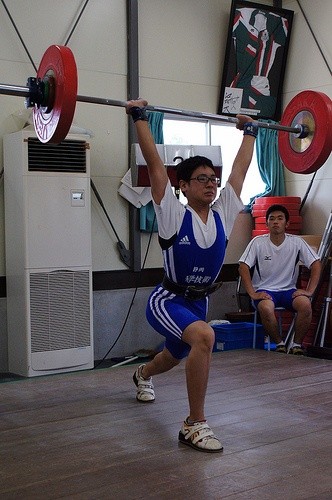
[126,97,258,453]
[238,204,323,354]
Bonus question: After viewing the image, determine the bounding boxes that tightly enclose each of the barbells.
[0,44,331,174]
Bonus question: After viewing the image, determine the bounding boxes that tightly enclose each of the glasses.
[185,174,221,185]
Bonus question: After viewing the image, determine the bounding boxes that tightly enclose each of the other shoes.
[290,345,303,356]
[276,343,287,353]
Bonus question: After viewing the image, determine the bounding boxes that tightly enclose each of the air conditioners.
[3,132,94,378]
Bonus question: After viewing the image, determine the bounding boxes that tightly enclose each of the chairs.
[236,267,295,350]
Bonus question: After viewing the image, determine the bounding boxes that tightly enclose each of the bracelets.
[129,105,148,121]
[243,122,258,137]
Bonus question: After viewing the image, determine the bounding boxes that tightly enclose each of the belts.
[161,273,224,299]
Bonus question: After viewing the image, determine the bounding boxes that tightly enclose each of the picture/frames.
[217,0,295,122]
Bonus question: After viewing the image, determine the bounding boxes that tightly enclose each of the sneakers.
[132,364,155,403]
[178,414,224,453]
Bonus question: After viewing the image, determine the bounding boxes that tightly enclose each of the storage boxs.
[297,235,323,255]
[211,322,263,352]
